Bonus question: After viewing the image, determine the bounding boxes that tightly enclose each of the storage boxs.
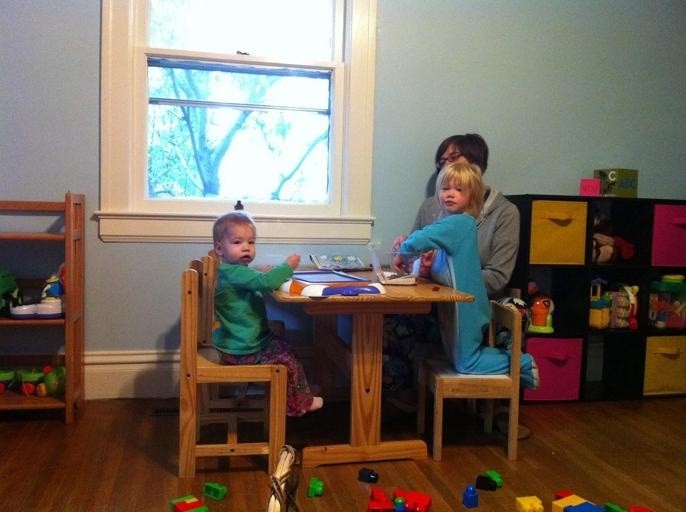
[523,337,583,401]
[529,200,587,266]
[651,204,686,268]
[644,337,686,397]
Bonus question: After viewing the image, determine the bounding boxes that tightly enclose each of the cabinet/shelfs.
[505,195,686,404]
[0,192,86,425]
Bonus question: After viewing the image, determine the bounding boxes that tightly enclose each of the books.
[308,253,366,271]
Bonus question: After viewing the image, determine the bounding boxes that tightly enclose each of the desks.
[269,264,474,470]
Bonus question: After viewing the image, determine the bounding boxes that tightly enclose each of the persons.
[392,132,534,441]
[391,159,541,393]
[210,210,327,417]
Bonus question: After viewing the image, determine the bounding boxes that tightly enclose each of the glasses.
[435,150,465,169]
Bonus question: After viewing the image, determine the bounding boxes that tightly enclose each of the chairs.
[417,300,522,461]
[201,249,286,413]
[179,259,287,478]
